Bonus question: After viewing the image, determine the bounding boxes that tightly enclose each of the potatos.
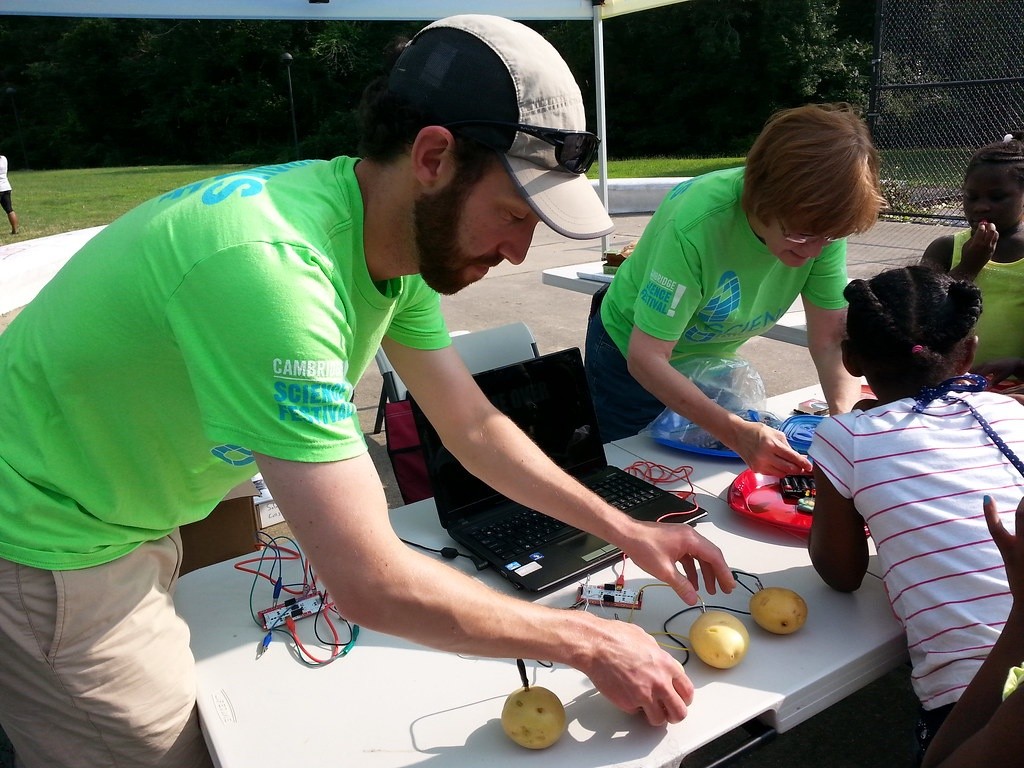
[689,610,749,669]
[502,686,566,749]
[749,587,808,634]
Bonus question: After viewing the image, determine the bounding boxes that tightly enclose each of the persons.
[0,11,739,768]
[916,488,1024,768]
[0,153,18,234]
[914,130,1024,372]
[582,101,891,480]
[805,264,1024,768]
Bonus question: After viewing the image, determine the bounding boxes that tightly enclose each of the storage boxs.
[178,478,263,578]
[252,487,287,529]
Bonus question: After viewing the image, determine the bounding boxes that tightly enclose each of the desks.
[173,262,971,768]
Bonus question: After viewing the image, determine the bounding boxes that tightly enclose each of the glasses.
[773,210,849,244]
[441,119,600,175]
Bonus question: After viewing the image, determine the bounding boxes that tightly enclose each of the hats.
[387,14,613,240]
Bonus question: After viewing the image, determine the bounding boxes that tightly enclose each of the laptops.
[408,347,708,592]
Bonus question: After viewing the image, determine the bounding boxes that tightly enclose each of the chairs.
[374,323,541,432]
